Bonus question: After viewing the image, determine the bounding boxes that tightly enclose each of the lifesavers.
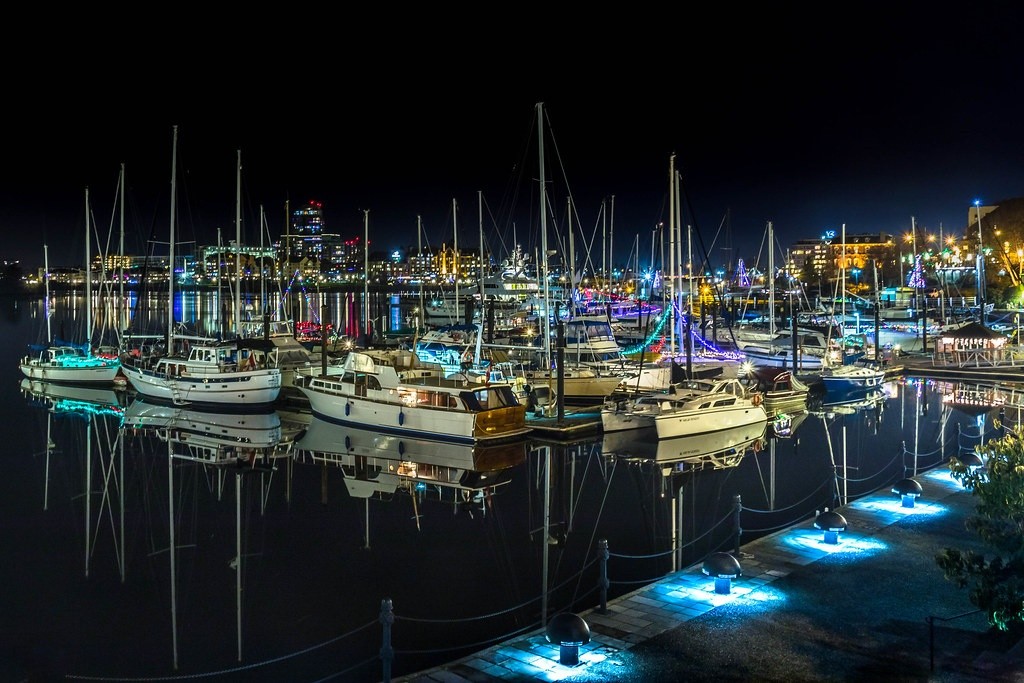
[754,395,760,405]
[754,440,761,452]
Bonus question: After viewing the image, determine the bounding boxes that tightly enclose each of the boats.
[291,309,534,442]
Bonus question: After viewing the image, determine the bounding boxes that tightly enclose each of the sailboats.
[96,163,1022,415]
[123,124,282,411]
[17,187,123,385]
[602,419,768,576]
[20,377,117,578]
[293,415,526,550]
[598,151,768,439]
[118,393,281,668]
